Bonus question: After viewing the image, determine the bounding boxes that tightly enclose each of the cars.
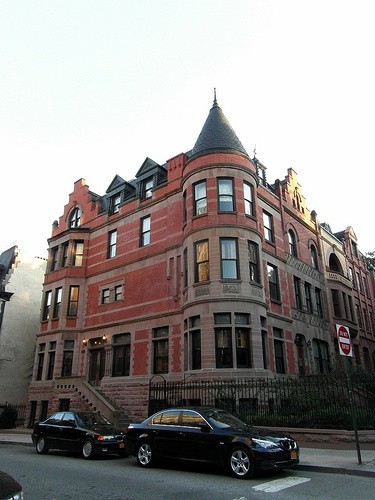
[126,407,299,479]
[31,411,126,460]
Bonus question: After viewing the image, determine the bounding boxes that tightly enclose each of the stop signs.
[336,324,353,357]
